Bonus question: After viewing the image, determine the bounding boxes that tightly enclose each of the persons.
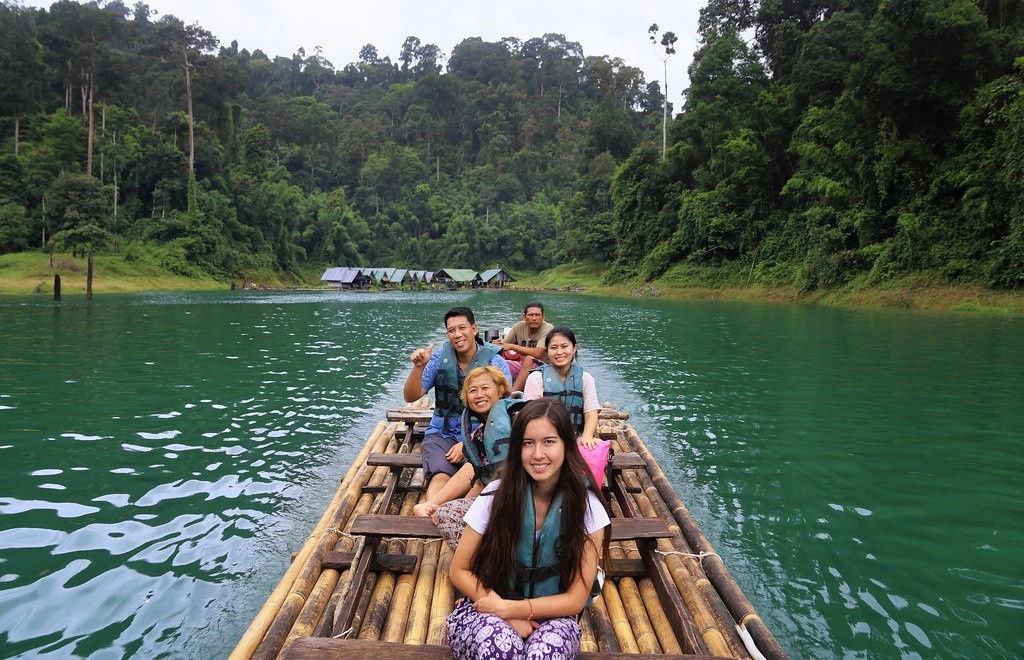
[434,365,534,553]
[492,303,554,393]
[446,397,613,660]
[404,306,514,525]
[524,327,602,451]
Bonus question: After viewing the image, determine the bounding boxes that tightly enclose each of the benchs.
[385,407,621,439]
[362,452,648,471]
[350,511,675,548]
[286,636,738,660]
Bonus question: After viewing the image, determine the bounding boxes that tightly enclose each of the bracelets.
[524,598,534,620]
